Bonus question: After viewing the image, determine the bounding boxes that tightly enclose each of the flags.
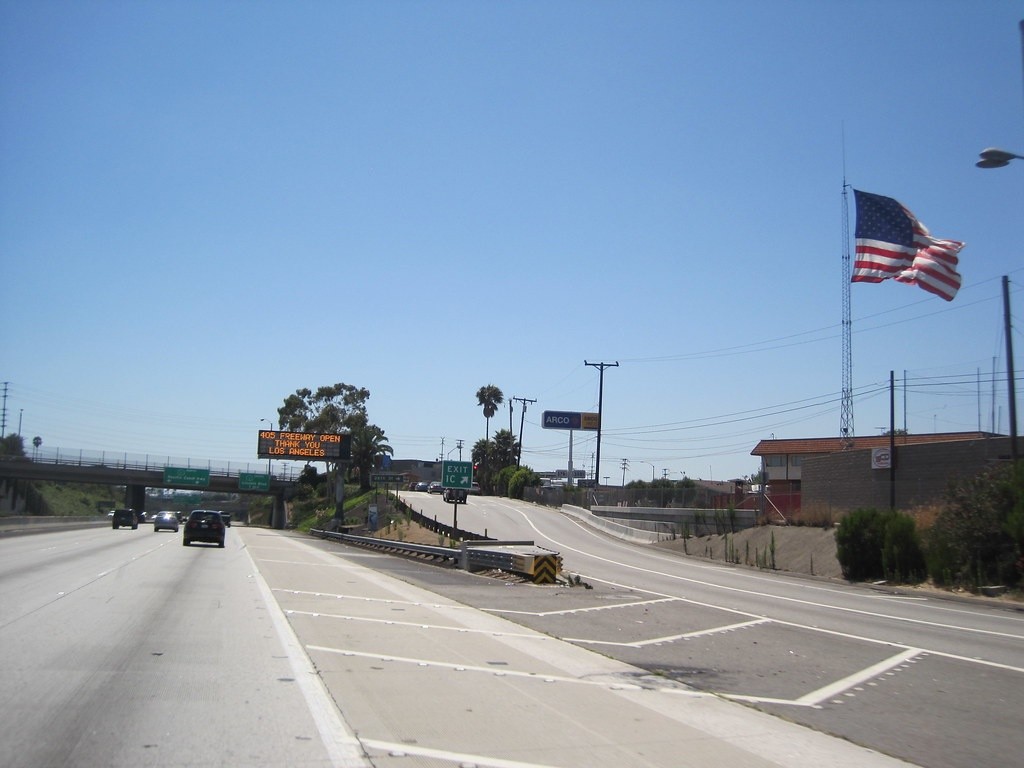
[850,189,966,302]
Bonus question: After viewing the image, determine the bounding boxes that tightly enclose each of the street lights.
[641,461,654,479]
[260,419,272,474]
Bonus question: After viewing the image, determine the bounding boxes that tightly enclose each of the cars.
[113,509,138,529]
[408,482,417,491]
[183,510,225,547]
[154,511,179,532]
[415,481,429,492]
[468,482,481,496]
[443,488,468,503]
[108,511,115,516]
[427,481,445,494]
